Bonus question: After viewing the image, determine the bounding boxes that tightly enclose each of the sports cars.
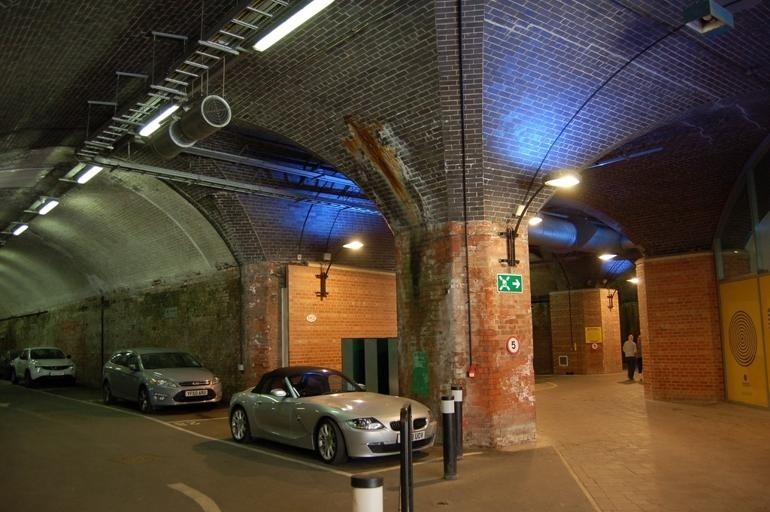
[226,367,436,465]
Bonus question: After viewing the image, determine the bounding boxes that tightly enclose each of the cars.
[99,347,222,415]
[8,346,75,388]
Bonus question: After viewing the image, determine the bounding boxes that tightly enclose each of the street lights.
[320,241,365,295]
[508,170,582,270]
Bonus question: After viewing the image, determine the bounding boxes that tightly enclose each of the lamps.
[671,0,735,42]
[72,162,105,185]
[499,169,582,266]
[251,0,335,52]
[315,239,365,302]
[607,277,641,313]
[138,103,180,137]
[11,223,29,236]
[36,198,59,216]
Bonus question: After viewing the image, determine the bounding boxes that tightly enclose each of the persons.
[623,334,638,380]
[636,335,642,376]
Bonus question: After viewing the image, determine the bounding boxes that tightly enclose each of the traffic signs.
[566,252,639,296]
[497,275,522,292]
